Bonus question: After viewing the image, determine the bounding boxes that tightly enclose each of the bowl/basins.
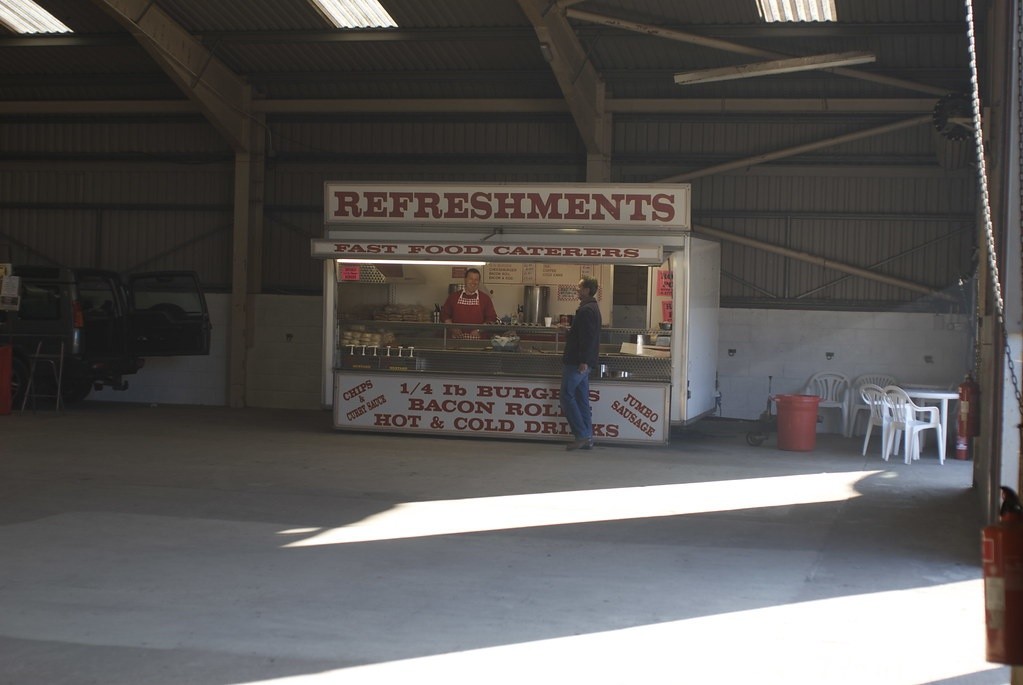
[658,321,673,330]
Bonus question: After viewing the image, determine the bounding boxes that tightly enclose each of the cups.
[544,316,553,328]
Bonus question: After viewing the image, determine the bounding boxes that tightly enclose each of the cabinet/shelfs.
[333,315,674,445]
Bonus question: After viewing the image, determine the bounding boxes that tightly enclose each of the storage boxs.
[522,283,551,326]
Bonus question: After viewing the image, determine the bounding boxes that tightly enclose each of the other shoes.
[567,438,593,449]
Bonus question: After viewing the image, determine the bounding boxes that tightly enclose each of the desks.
[897,384,950,448]
[904,389,959,464]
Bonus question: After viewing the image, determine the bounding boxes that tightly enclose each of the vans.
[0,260,215,414]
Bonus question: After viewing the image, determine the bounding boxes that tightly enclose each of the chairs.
[859,383,915,460]
[849,373,899,439]
[806,370,850,438]
[881,384,945,465]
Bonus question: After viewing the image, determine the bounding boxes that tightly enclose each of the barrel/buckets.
[517,283,550,325]
[775,392,822,453]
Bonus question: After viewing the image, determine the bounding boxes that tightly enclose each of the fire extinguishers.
[981,486,1022,666]
[958,370,980,436]
[956,435,970,460]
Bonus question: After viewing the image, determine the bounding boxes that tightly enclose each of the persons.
[439,268,497,347]
[560,276,602,451]
